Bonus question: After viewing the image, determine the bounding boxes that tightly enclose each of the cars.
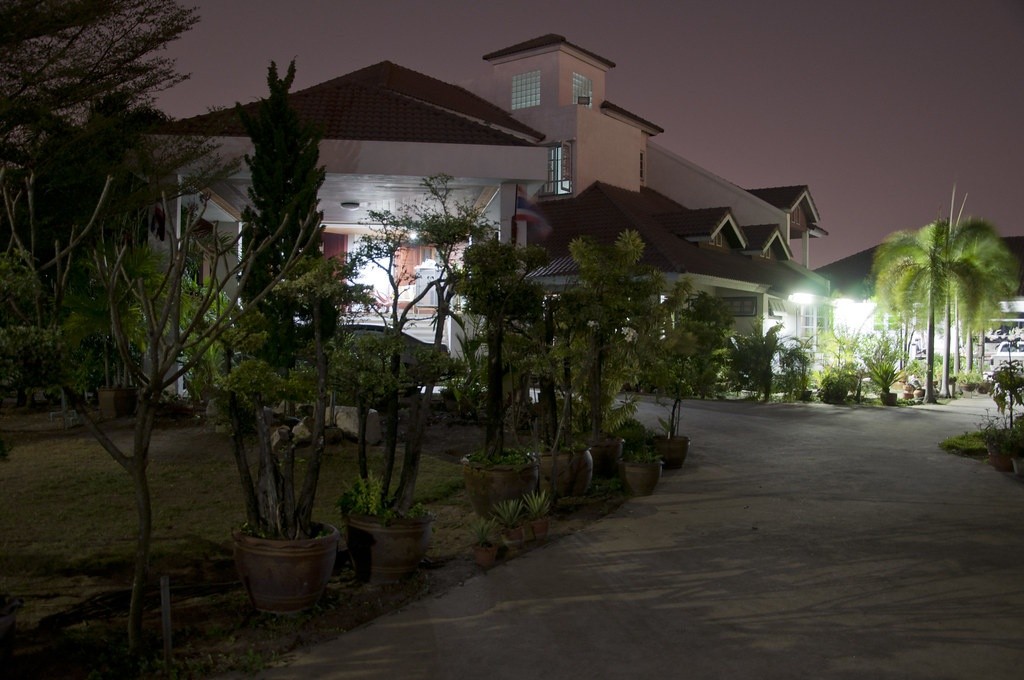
[333,323,449,397]
[988,341,1024,380]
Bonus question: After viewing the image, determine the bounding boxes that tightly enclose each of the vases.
[793,389,812,399]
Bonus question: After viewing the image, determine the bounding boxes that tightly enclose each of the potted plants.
[976,407,1024,477]
[523,490,551,540]
[56,240,192,418]
[868,360,925,406]
[488,498,524,550]
[440,226,716,519]
[959,370,991,394]
[325,173,500,582]
[469,516,505,566]
[192,255,374,617]
